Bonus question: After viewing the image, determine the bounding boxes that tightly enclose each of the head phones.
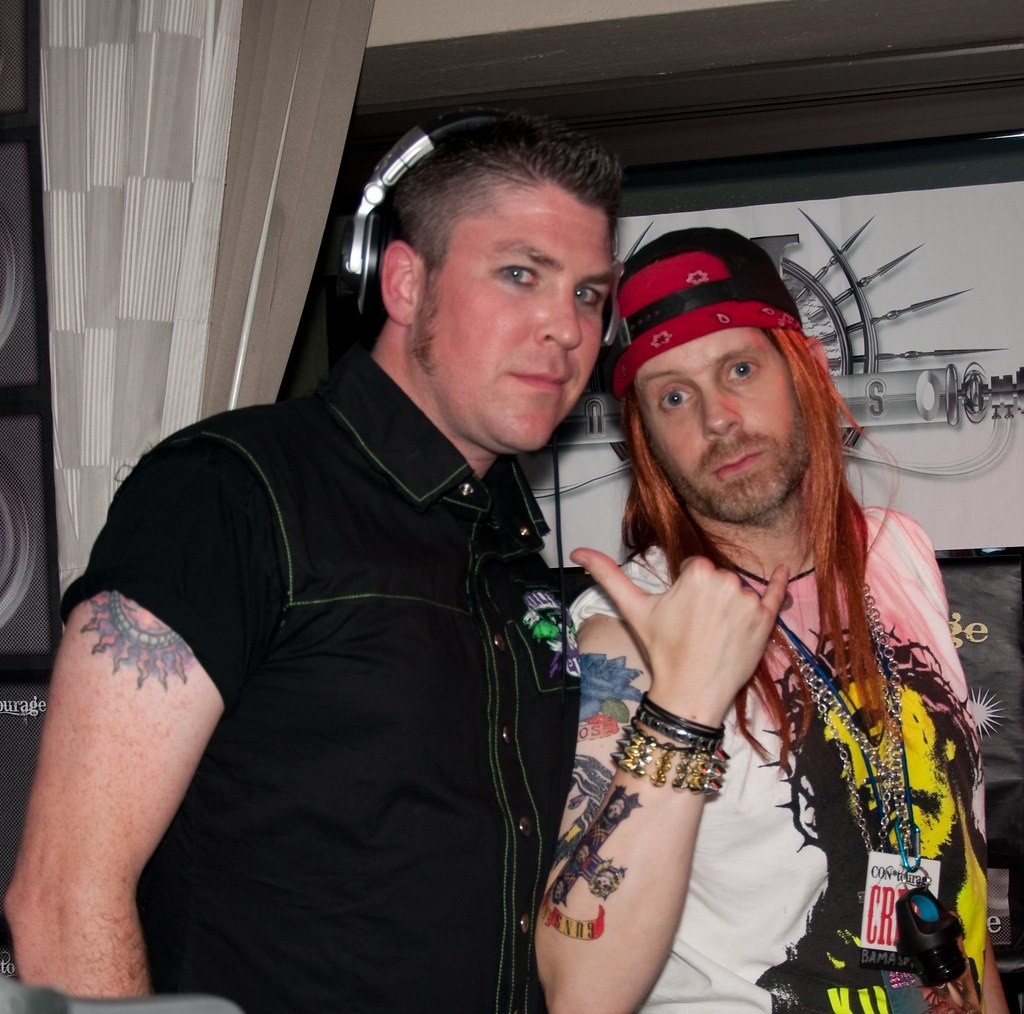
[339,110,624,346]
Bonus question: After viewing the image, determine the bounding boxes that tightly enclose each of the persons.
[3,103,626,1014]
[531,225,1011,1014]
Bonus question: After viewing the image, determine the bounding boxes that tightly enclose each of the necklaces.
[769,583,925,973]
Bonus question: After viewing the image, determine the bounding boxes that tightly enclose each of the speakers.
[0,0,66,979]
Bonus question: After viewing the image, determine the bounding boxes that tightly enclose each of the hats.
[613,252,800,399]
[601,228,800,391]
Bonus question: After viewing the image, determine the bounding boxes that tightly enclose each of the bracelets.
[614,728,732,796]
[627,691,727,754]
